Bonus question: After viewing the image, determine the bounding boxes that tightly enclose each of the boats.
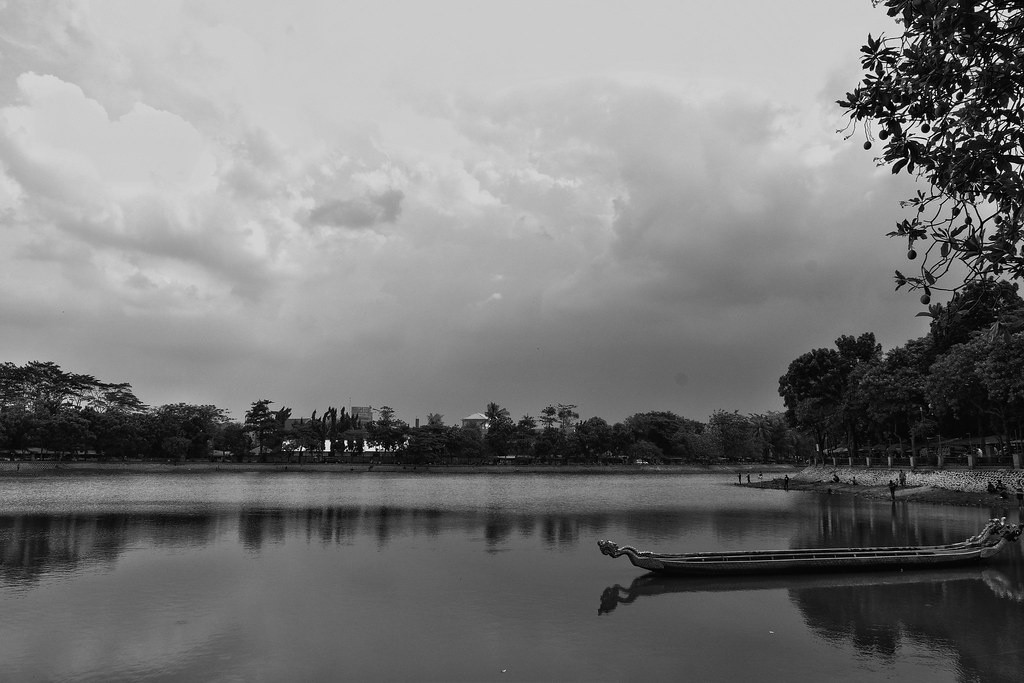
[597,516,1024,577]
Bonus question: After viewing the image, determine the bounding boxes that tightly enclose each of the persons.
[986,479,1010,502]
[737,469,907,503]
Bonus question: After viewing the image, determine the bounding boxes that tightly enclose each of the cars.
[634,459,649,466]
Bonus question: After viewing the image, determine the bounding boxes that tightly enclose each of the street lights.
[966,433,973,455]
[936,434,941,455]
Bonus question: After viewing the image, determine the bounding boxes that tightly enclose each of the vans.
[324,457,341,463]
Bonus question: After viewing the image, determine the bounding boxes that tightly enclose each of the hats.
[889,480,893,482]
[997,479,1003,482]
